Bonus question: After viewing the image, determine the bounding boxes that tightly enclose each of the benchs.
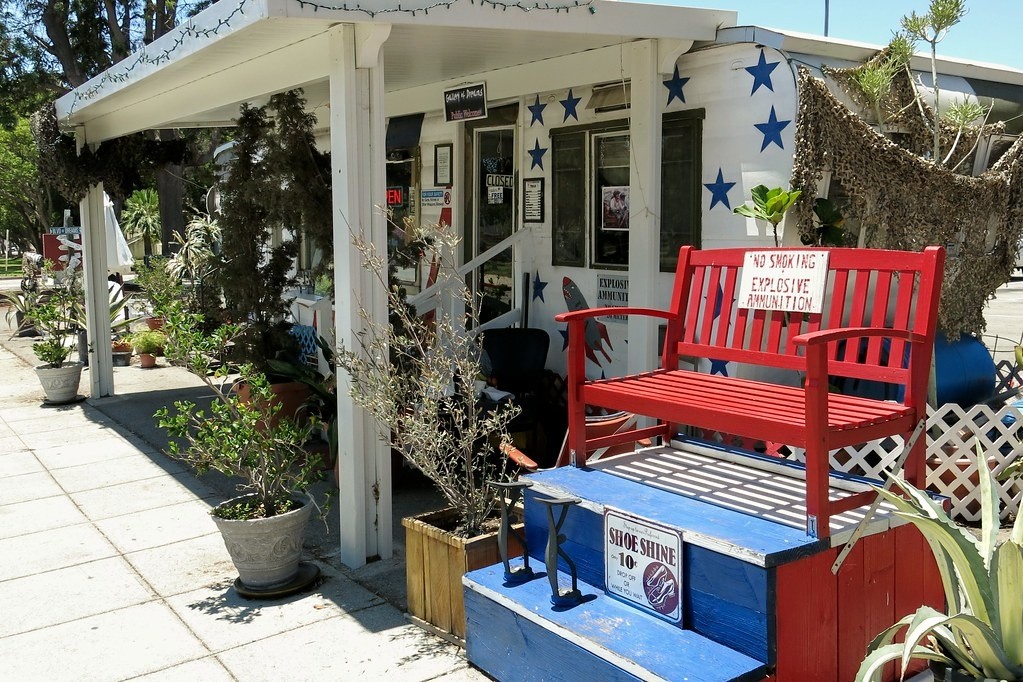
[554,246,948,538]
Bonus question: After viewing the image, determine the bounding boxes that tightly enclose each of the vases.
[112,352,132,366]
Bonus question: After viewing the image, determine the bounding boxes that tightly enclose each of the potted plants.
[0,290,52,338]
[23,254,84,402]
[131,254,183,330]
[325,202,528,650]
[134,330,165,368]
[152,312,328,592]
[198,203,315,461]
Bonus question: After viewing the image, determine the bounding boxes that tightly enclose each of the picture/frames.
[522,177,545,223]
[433,143,454,187]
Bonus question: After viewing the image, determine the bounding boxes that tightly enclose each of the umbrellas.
[103,191,141,331]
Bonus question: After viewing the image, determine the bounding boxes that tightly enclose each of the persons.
[610,190,627,227]
